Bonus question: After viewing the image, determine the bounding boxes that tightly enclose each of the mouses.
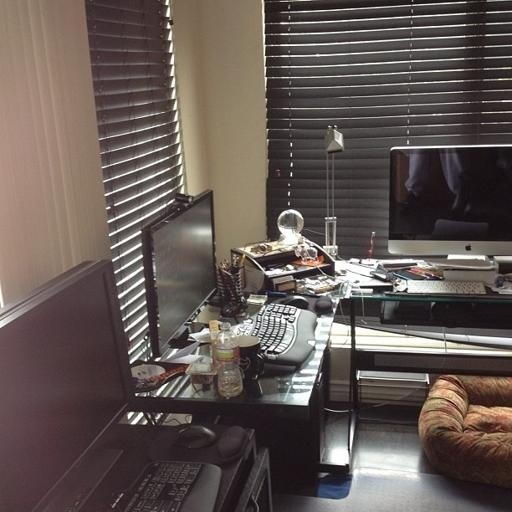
[280,296,309,309]
[176,425,215,448]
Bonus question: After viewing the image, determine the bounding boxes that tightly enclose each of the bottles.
[213,320,243,398]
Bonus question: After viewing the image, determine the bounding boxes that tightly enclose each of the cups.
[233,334,265,384]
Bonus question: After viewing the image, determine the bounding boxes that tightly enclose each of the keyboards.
[407,280,486,295]
[248,303,315,366]
[110,460,222,512]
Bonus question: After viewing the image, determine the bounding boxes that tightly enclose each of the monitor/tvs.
[1,257,132,512]
[388,144,512,269]
[143,190,217,357]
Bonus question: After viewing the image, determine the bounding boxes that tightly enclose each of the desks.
[348,257,512,451]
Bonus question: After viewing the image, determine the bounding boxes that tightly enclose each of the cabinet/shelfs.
[37,423,273,512]
[128,292,339,497]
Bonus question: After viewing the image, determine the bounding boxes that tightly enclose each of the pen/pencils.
[217,253,246,305]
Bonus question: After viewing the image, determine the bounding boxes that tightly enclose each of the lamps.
[322,124,346,261]
